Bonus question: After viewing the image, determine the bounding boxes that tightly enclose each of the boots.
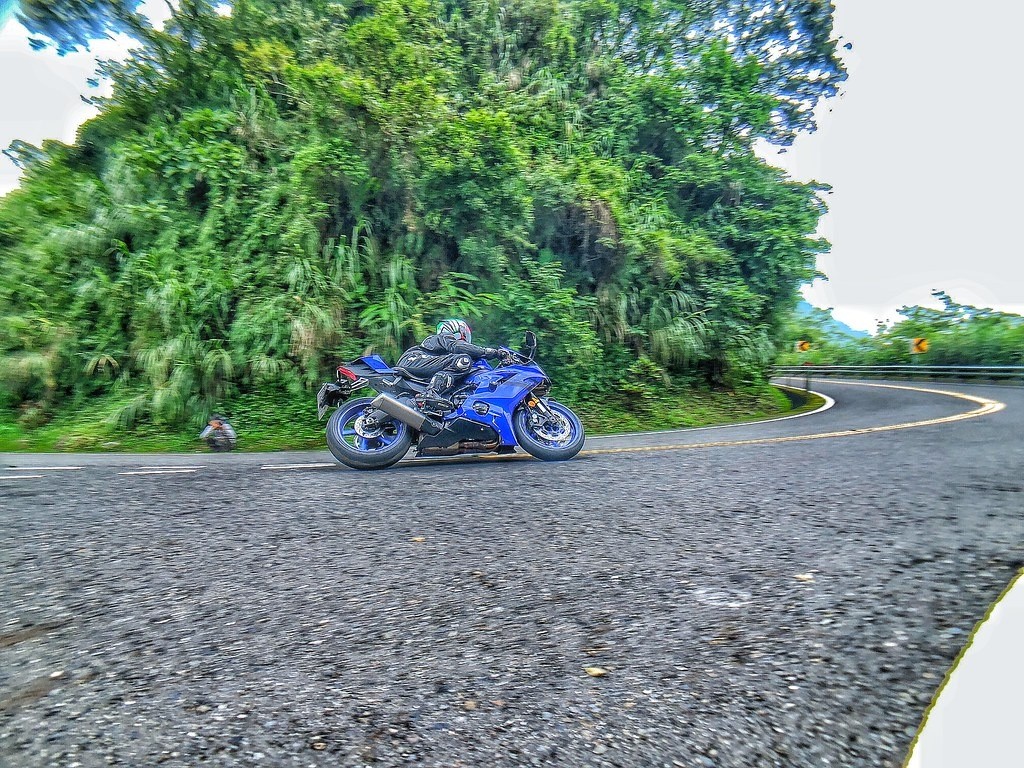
[414,371,454,410]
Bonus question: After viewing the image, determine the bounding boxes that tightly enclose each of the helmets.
[437,319,471,344]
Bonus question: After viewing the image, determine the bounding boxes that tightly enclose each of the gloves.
[485,347,510,359]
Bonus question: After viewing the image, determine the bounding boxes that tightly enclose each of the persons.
[397,319,513,411]
[199,413,237,454]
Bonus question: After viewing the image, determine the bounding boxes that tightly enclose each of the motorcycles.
[316,330,585,471]
